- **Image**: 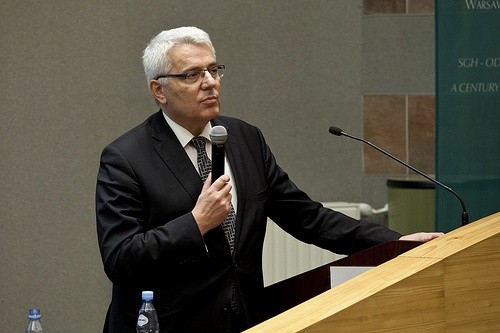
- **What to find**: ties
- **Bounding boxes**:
[188,135,242,315]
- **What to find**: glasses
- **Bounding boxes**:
[155,63,225,83]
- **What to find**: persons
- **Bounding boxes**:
[95,26,445,333]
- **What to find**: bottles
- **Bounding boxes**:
[137,291,160,333]
[24,309,43,333]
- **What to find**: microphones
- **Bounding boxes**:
[329,126,469,226]
[209,125,228,187]
